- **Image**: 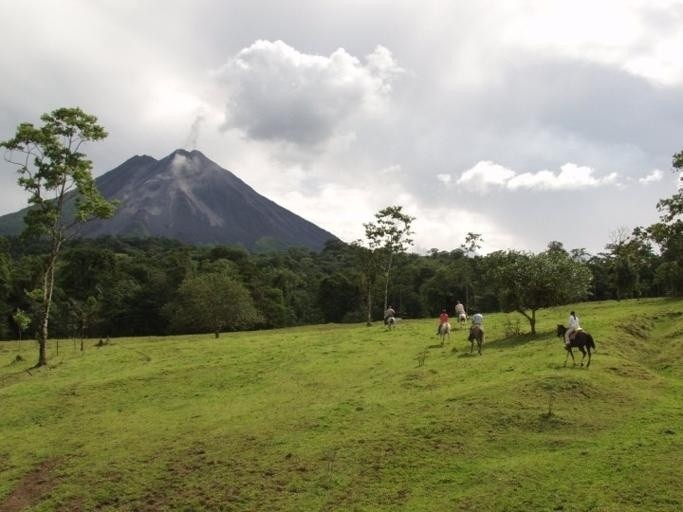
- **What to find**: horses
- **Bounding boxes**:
[556,323,595,368]
[439,322,451,347]
[384,310,395,330]
[458,313,466,330]
[468,318,484,355]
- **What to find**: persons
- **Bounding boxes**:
[455,299,465,322]
[438,309,449,333]
[384,305,395,320]
[565,310,579,344]
[473,311,483,325]
